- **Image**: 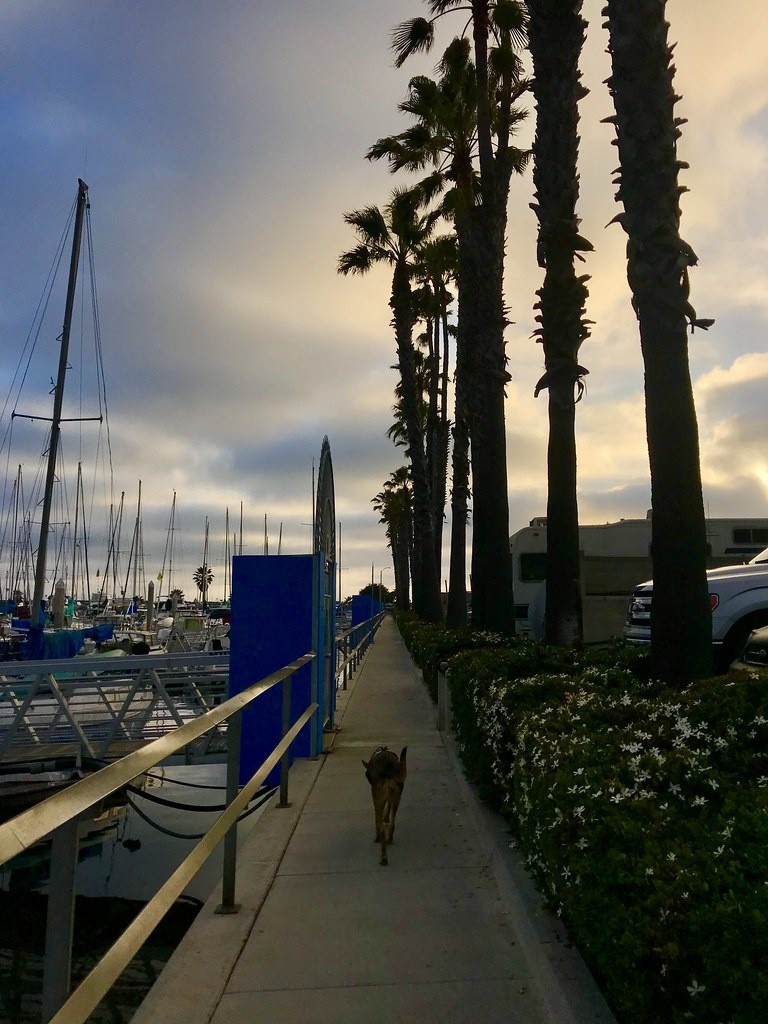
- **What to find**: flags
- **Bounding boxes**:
[96,569,99,577]
[157,572,163,581]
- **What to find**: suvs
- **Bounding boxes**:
[621,545,768,676]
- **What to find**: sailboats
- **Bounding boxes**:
[0,161,230,863]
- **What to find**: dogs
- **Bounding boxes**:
[362,745,408,867]
